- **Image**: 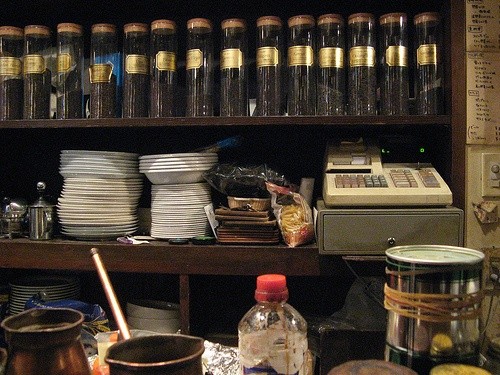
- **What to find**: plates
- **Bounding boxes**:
[56,150,143,239]
[9,279,79,315]
[150,185,215,240]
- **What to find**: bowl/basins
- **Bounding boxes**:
[125,298,180,333]
[138,152,219,184]
[227,196,270,211]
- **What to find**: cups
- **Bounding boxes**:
[26,198,56,240]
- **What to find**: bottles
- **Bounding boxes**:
[381,12,409,118]
[414,12,446,116]
[23,24,51,121]
[286,15,317,116]
[346,12,377,117]
[149,19,179,118]
[314,15,345,118]
[90,24,117,119]
[185,18,215,118]
[219,19,251,117]
[255,15,286,116]
[55,23,85,121]
[0,26,25,121]
[0,307,93,375]
[123,23,148,119]
[104,333,206,375]
[237,274,310,375]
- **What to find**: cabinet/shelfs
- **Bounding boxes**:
[0,0,466,336]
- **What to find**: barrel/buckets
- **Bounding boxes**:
[382,245,486,375]
